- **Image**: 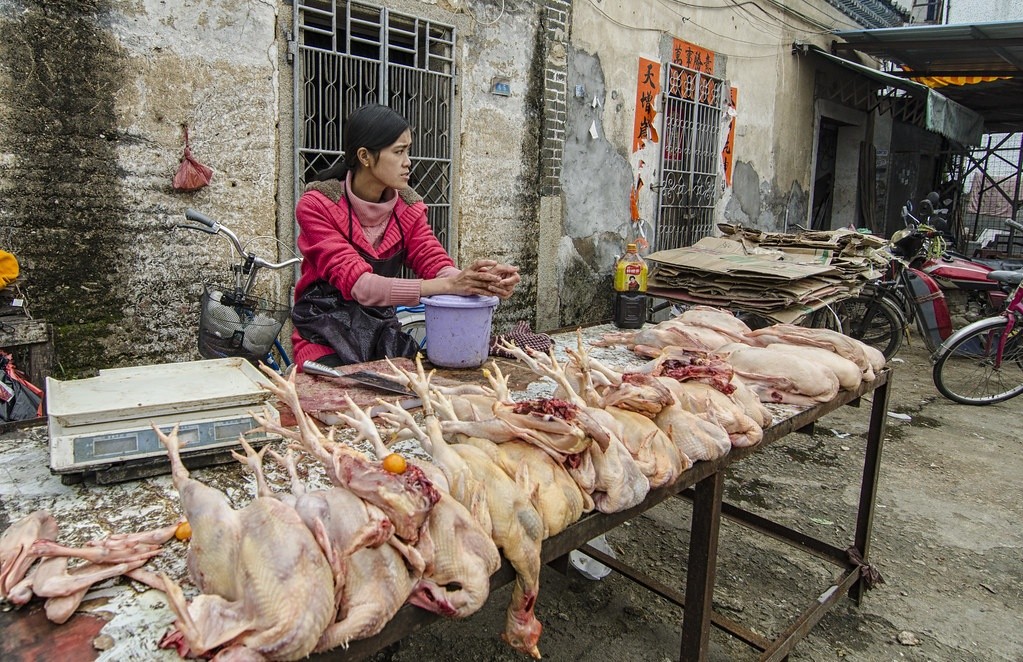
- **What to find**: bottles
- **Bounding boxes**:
[615,243,648,328]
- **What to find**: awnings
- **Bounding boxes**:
[791,39,986,150]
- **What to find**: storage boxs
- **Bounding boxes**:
[419,290,500,372]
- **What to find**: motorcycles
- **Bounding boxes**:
[843,191,1023,361]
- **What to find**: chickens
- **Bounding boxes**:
[148,328,773,658]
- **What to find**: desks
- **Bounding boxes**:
[0,311,893,662]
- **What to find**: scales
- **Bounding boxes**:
[45,356,283,486]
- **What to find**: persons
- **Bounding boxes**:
[287,105,523,375]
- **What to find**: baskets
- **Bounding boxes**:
[197,284,291,369]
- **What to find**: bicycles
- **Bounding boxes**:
[177,207,428,375]
[928,217,1023,405]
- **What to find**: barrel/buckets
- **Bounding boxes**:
[420,292,498,368]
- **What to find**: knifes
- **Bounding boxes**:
[302,360,418,399]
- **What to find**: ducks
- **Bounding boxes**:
[767,340,862,392]
[743,323,886,382]
[587,321,727,359]
[712,342,841,406]
[673,304,753,347]
